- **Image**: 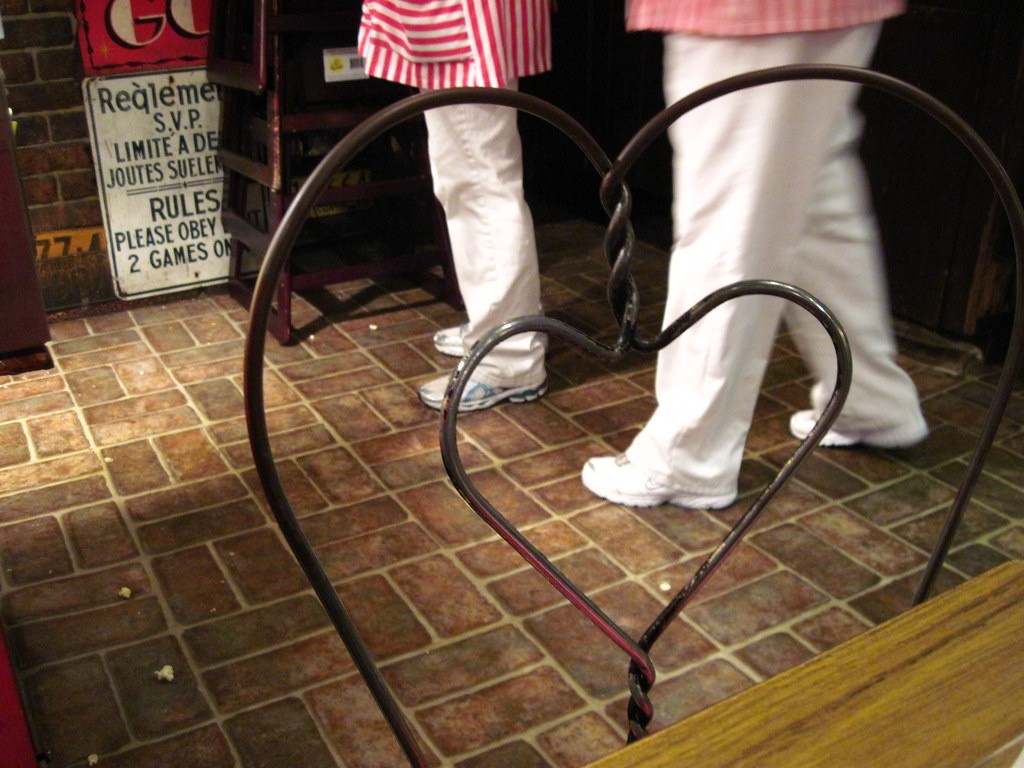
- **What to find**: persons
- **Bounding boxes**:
[581,0,930,510]
[358,0,552,412]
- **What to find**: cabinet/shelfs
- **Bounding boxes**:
[208,0,464,345]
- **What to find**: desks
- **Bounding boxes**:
[596,560,1024,768]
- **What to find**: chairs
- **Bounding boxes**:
[245,61,1024,768]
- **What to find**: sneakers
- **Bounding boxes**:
[420,369,548,413]
[790,409,930,449]
[581,454,737,510]
[434,322,469,357]
